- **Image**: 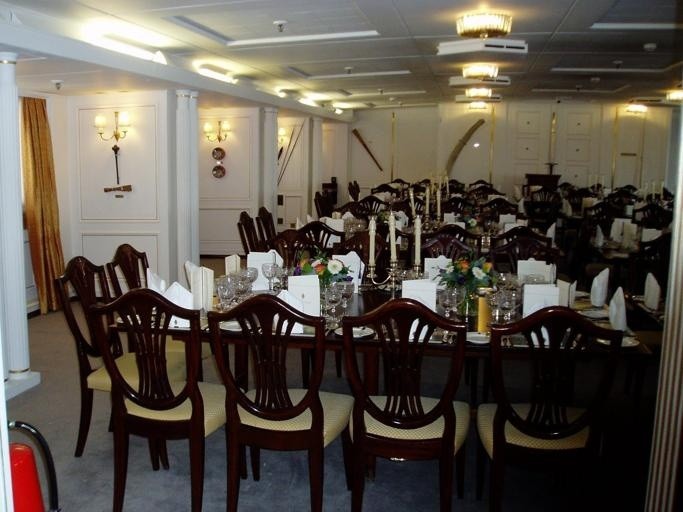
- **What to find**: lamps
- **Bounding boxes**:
[203,120,230,143]
[463,63,498,109]
[94,113,130,155]
[455,12,513,40]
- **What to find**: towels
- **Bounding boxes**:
[162,282,194,328]
[146,268,165,296]
[185,260,214,311]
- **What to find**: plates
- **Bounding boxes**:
[218,282,638,347]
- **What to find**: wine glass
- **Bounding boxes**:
[215,261,547,346]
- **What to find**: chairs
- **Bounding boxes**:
[106,244,212,359]
[53,255,187,469]
[86,288,245,512]
[342,297,473,512]
[206,293,355,511]
[7,420,63,512]
[473,305,623,512]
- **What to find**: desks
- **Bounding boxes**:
[525,173,561,193]
